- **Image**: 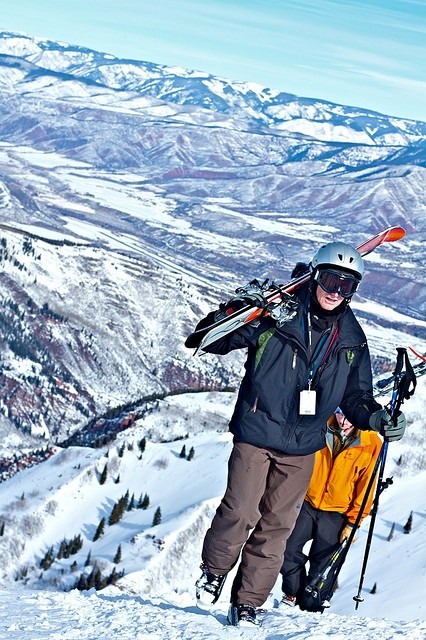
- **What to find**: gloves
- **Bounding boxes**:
[368,408,406,442]
[213,289,269,322]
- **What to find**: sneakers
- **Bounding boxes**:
[195,562,227,607]
[277,594,297,610]
[227,603,264,627]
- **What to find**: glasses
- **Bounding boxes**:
[309,262,361,298]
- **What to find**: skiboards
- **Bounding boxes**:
[184,226,406,356]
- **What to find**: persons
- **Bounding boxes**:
[195,242,407,630]
[279,407,383,615]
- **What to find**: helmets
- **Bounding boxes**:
[308,242,365,281]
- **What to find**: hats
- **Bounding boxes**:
[333,407,344,416]
